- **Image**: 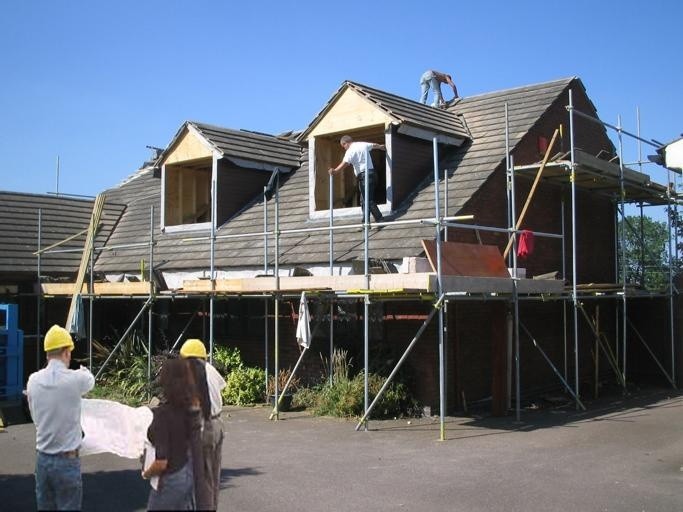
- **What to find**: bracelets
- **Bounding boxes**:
[141,472,149,480]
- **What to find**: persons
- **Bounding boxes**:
[418,70,459,111]
[328,134,386,232]
[26,324,95,511]
[182,359,211,435]
[179,338,226,512]
[139,357,203,511]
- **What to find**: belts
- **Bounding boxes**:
[50,450,80,457]
[211,414,221,419]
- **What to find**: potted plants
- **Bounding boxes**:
[268,369,301,412]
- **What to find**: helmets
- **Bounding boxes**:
[44,324,75,353]
[180,338,206,358]
